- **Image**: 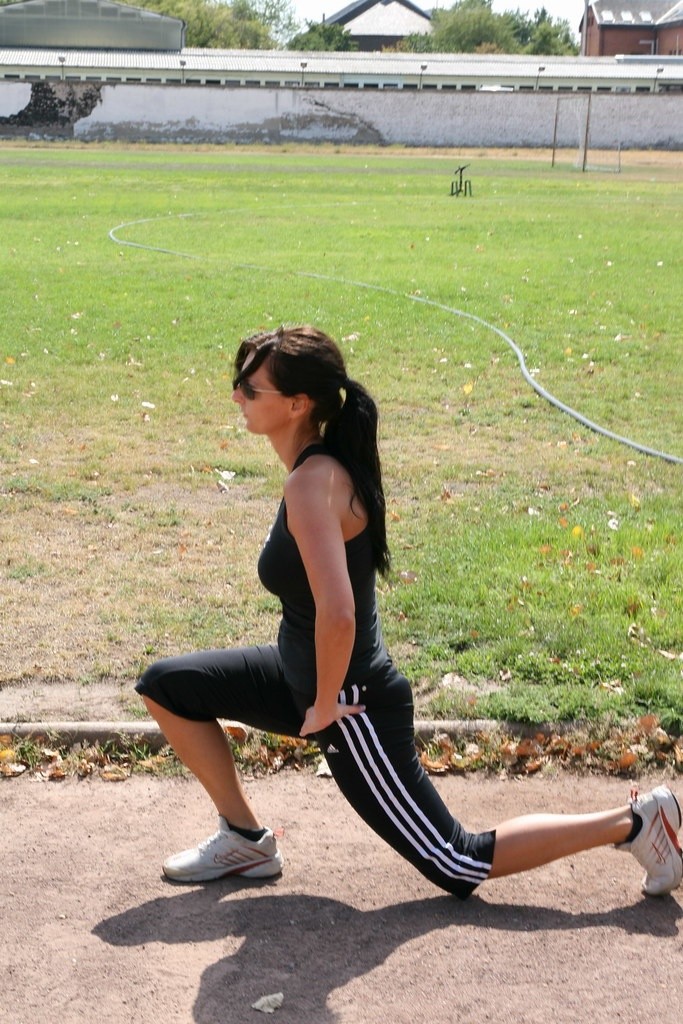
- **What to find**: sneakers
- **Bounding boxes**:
[162,815,284,881]
[615,785,683,896]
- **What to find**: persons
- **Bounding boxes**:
[135,328,683,896]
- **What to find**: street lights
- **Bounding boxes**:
[417,62,429,91]
[534,66,545,91]
[300,61,307,88]
[58,56,68,81]
[179,60,187,84]
[651,67,664,95]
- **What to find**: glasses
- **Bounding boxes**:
[239,380,282,400]
